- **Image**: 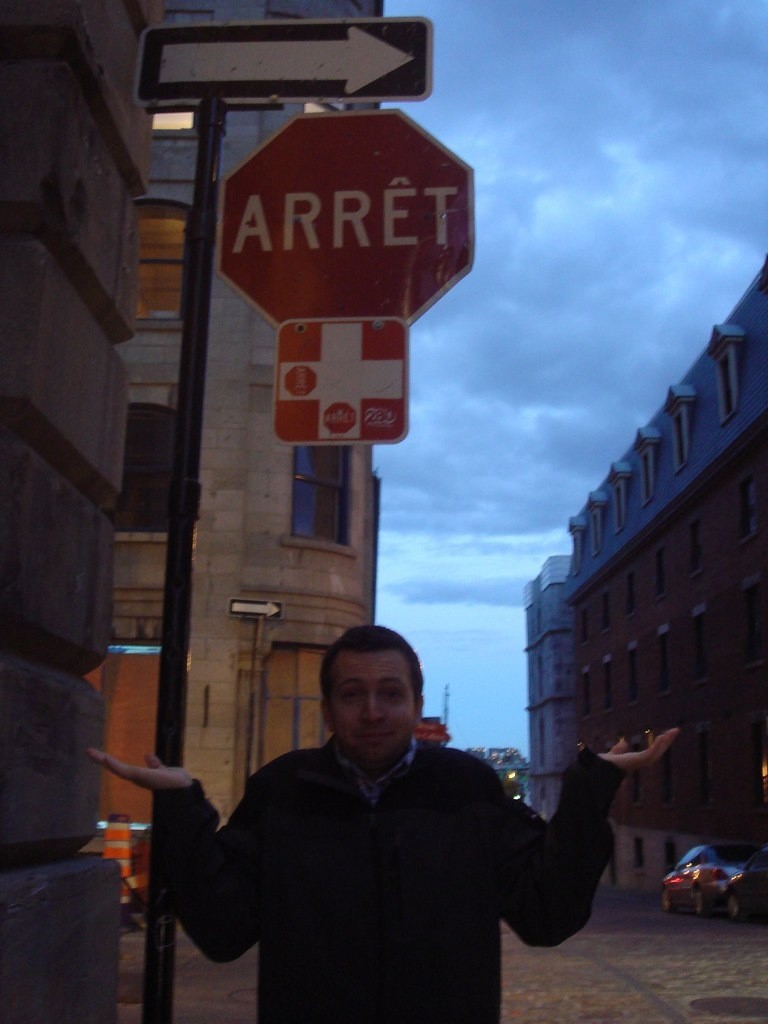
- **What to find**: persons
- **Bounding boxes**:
[84,624,678,1024]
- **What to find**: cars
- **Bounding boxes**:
[720,846,768,923]
[661,842,760,917]
